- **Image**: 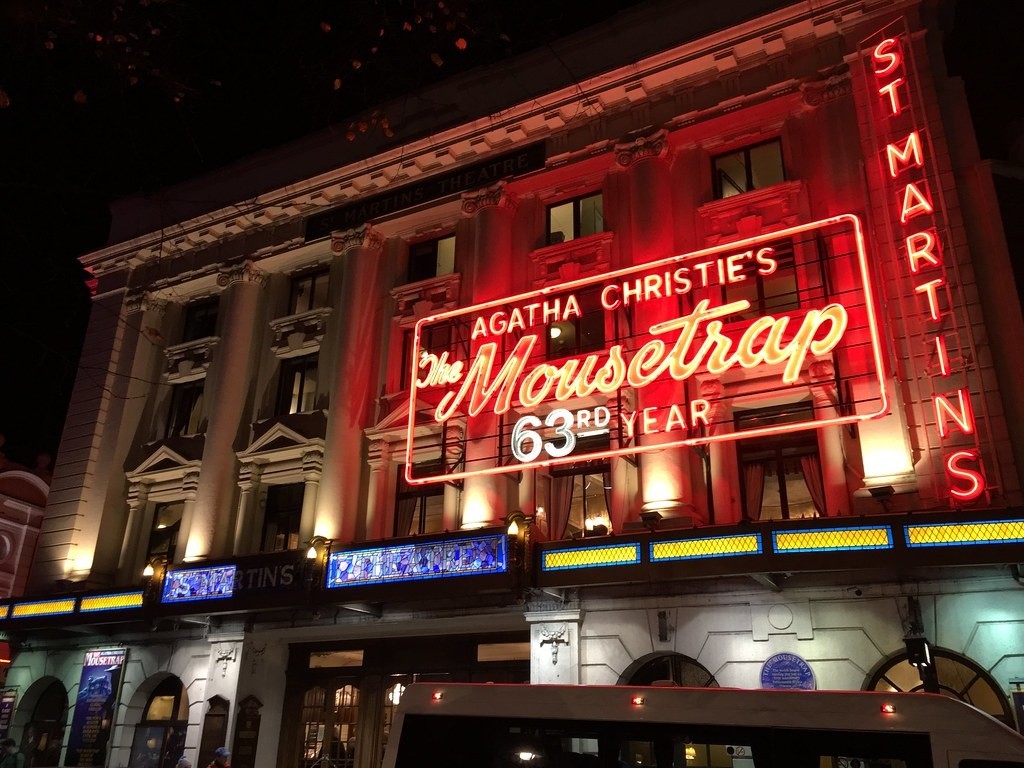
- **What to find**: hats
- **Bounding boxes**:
[215,747,231,756]
[178,755,192,768]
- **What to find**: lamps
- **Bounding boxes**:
[868,484,895,511]
[305,536,328,565]
[507,512,523,562]
[640,511,663,529]
[901,634,931,682]
[142,557,163,576]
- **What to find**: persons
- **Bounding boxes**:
[176,756,192,768]
[20,727,61,768]
[316,727,388,768]
[0,738,25,768]
[207,747,231,768]
